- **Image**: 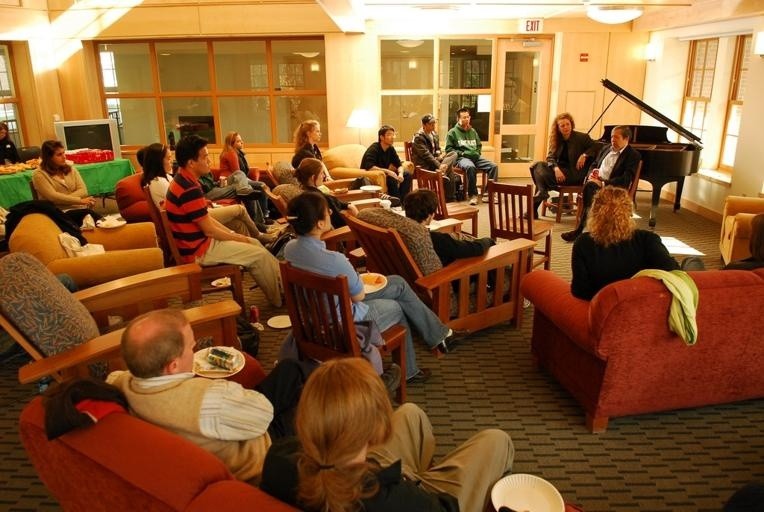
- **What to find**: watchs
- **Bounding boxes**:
[602,181,605,187]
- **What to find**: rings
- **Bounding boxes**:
[559,178,560,180]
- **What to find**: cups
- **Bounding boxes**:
[219,176,224,188]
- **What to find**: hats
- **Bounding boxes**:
[422,115,439,124]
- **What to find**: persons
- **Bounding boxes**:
[519,113,599,218]
[2,107,514,511]
[561,124,640,241]
[570,185,681,301]
[681,214,764,272]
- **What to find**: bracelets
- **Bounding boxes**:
[582,153,587,158]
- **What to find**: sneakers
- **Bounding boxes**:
[482,193,498,204]
[379,363,400,391]
[469,195,478,205]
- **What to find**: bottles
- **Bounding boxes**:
[64,147,114,164]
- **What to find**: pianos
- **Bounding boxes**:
[585,79,704,231]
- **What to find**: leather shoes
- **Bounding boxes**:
[519,211,538,220]
[438,329,469,353]
[407,368,432,383]
[561,230,576,240]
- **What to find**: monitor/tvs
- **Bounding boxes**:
[53,119,122,161]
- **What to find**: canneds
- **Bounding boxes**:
[207,349,237,370]
[592,169,599,179]
[249,306,260,323]
[219,176,225,188]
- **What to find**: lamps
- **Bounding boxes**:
[752,30,764,57]
[646,42,656,62]
[583,4,644,26]
[346,109,373,143]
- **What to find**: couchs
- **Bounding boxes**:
[16,388,587,511]
[516,259,761,435]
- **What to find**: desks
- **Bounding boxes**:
[658,233,707,257]
[0,158,134,210]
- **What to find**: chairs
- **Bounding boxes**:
[575,159,643,227]
[278,258,411,403]
[486,178,553,273]
[8,214,167,286]
[0,247,241,385]
[542,141,602,221]
[718,193,764,265]
[113,143,413,226]
[414,165,486,239]
[142,183,247,317]
[320,225,358,258]
[338,210,538,361]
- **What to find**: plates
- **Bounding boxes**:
[193,346,246,379]
[210,277,231,287]
[267,314,292,329]
[360,273,388,294]
[491,472,566,512]
[97,220,128,229]
[360,185,383,192]
